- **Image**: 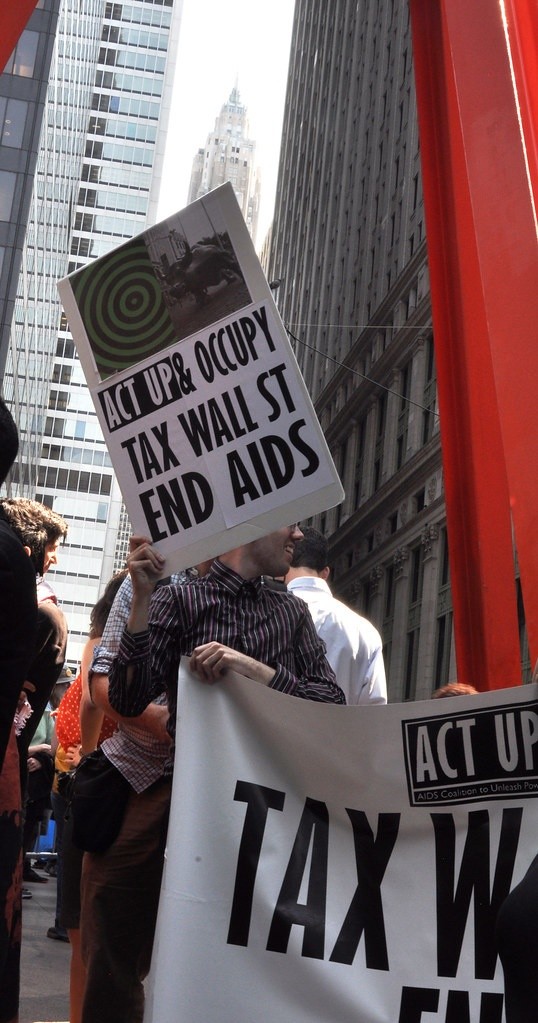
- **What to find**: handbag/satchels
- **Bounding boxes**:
[63,749,131,850]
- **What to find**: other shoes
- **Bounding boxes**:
[22,888,32,898]
[47,927,71,945]
[22,867,49,883]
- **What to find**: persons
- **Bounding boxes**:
[48,560,130,1023]
[42,667,75,718]
[109,524,349,758]
[0,498,66,828]
[0,401,37,778]
[22,708,56,882]
[279,527,388,705]
[81,555,214,1023]
[20,498,67,663]
